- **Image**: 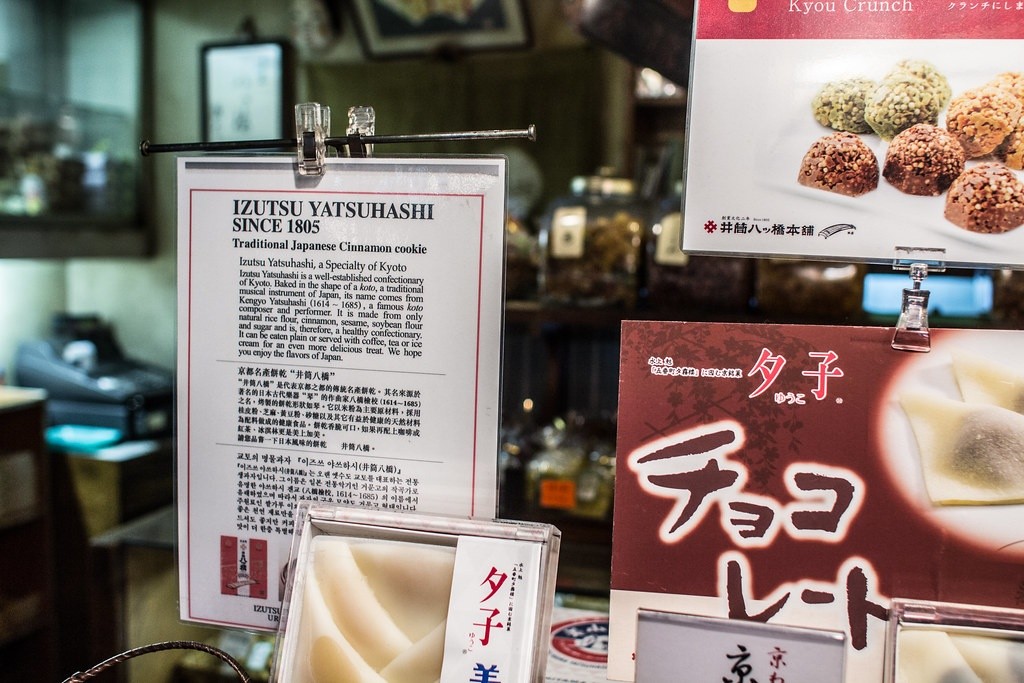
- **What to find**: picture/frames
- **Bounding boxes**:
[346,0,539,62]
[197,36,296,142]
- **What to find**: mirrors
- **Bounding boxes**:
[0,0,159,265]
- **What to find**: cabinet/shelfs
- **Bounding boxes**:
[0,384,60,683]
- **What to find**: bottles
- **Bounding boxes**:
[539,173,642,308]
[647,182,758,306]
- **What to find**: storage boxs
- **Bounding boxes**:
[68,438,173,532]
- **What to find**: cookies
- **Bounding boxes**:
[797,58,1024,234]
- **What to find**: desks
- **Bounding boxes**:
[506,297,856,418]
[87,508,177,683]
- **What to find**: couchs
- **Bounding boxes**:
[17,312,175,439]
